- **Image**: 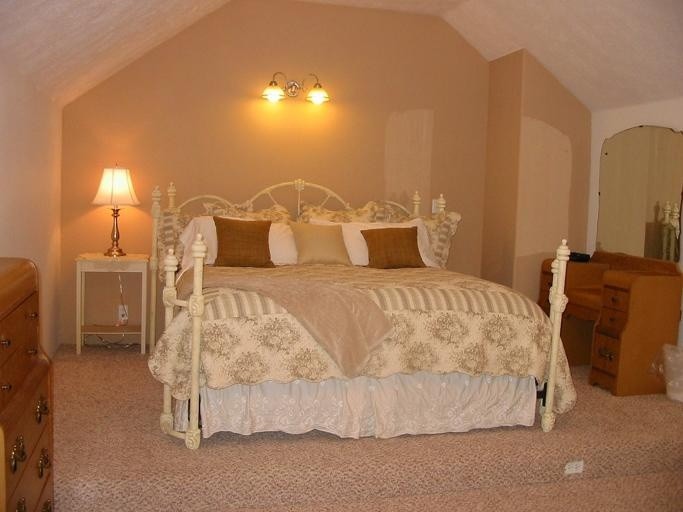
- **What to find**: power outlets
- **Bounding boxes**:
[118,305,128,320]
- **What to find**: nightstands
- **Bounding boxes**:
[75,254,150,355]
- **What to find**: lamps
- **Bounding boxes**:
[91,161,141,256]
[261,72,331,105]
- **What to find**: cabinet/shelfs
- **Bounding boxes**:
[0,258,54,512]
[537,249,683,396]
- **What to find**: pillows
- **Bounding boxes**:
[180,214,436,267]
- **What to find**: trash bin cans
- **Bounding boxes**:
[661,344,683,403]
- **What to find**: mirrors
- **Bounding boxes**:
[596,125,683,266]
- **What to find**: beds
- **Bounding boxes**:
[150,179,577,449]
